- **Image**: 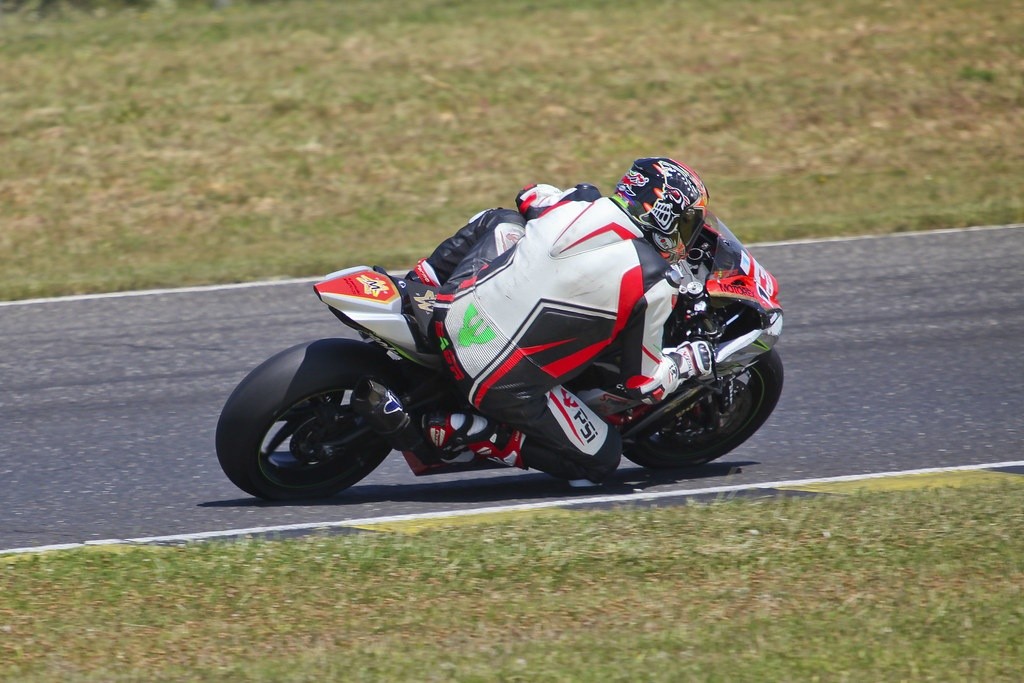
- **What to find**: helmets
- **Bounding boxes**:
[610,156,709,264]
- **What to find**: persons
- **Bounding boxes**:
[406,156,715,492]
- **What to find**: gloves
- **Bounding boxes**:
[666,337,719,384]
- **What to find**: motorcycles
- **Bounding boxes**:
[212,204,786,502]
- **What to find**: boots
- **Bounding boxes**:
[421,408,529,472]
[405,257,440,287]
